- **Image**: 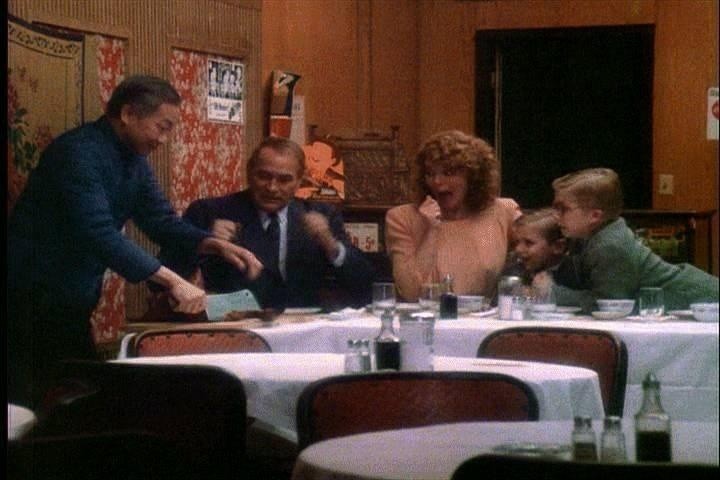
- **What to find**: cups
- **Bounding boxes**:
[372,281,395,319]
[639,287,665,320]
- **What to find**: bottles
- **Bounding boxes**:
[344,305,434,373]
[573,370,671,461]
[512,296,529,321]
[497,275,522,320]
[440,272,458,320]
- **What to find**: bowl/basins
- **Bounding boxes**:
[690,303,720,322]
[455,294,485,312]
[597,299,635,317]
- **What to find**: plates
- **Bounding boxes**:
[591,311,625,320]
[279,307,321,315]
[492,442,566,460]
[667,310,693,317]
[530,304,582,318]
[377,302,423,311]
[331,306,366,319]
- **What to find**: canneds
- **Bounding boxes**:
[344,339,371,375]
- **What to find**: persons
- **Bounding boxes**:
[384,130,526,302]
[1,75,265,437]
[294,138,346,199]
[146,135,373,323]
[489,207,585,307]
[531,166,720,314]
[209,62,242,99]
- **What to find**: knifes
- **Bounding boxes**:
[174,289,263,323]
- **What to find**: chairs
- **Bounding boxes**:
[451,453,720,479]
[296,373,538,448]
[7,362,248,480]
[127,325,271,357]
[477,327,627,416]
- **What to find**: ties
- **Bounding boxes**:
[265,210,280,273]
